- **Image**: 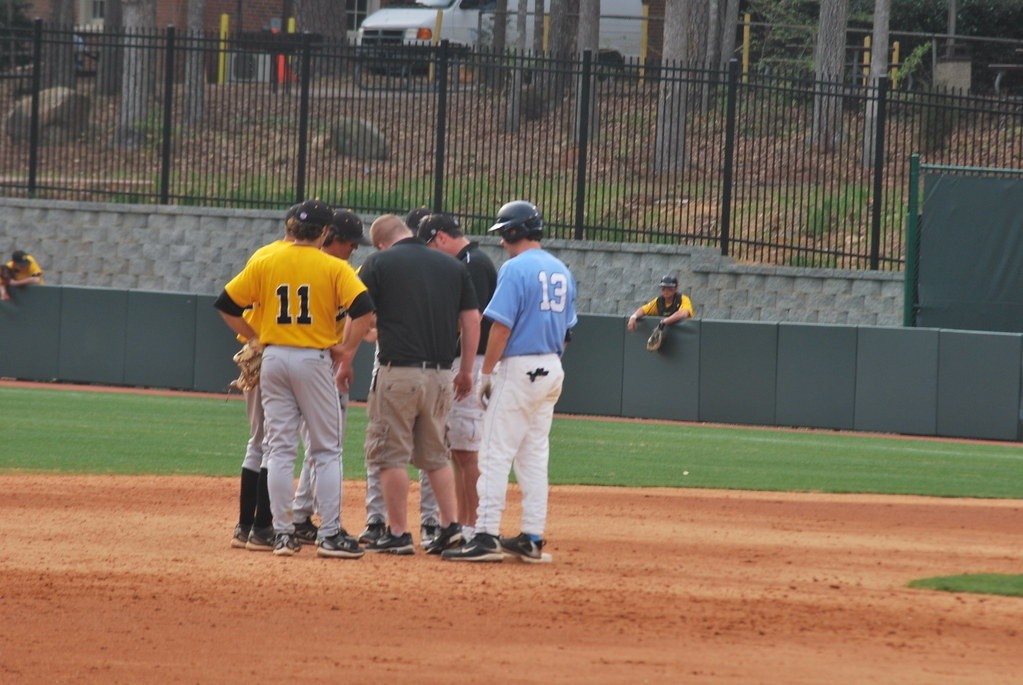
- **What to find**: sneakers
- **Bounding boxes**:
[421,524,440,547]
[272,533,301,555]
[293,516,318,544]
[359,521,385,543]
[501,532,544,561]
[231,522,250,547]
[364,525,415,554]
[425,522,466,554]
[442,533,503,562]
[245,523,276,552]
[316,529,364,559]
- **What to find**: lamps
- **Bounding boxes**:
[222,52,272,84]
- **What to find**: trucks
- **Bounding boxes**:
[355,0,643,83]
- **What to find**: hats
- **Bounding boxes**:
[12,251,32,266]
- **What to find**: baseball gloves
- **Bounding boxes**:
[224,334,263,403]
[646,320,665,352]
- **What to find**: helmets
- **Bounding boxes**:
[487,200,538,233]
[295,201,332,224]
[417,214,453,245]
[406,207,432,236]
[332,209,371,247]
[286,203,301,224]
[659,275,677,287]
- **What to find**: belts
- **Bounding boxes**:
[380,360,451,370]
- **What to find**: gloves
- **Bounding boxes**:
[526,368,548,382]
[476,369,492,410]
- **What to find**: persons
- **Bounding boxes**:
[0,266,12,287]
[627,275,693,350]
[440,202,578,562]
[358,208,439,544]
[214,201,376,561]
[357,214,482,556]
[0,250,44,300]
[417,214,498,550]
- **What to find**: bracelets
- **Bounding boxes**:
[658,321,664,330]
[630,315,637,320]
